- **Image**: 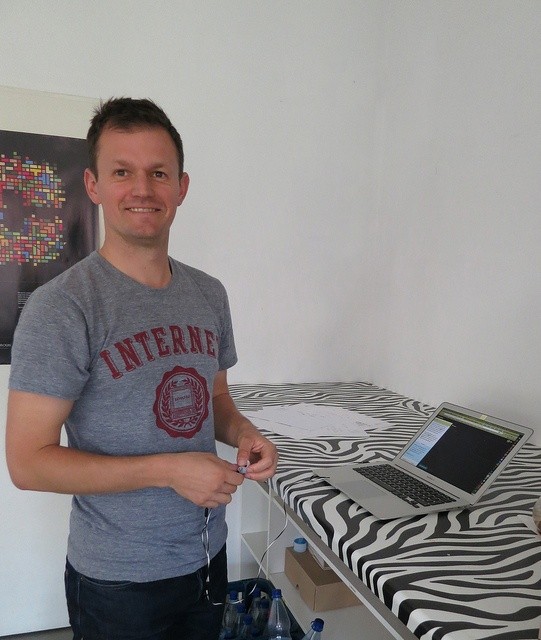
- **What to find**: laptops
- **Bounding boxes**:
[311,401,535,521]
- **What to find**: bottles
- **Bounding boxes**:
[269,589,291,640]
[224,590,243,630]
[234,604,246,640]
[248,588,268,634]
[249,629,264,640]
[219,594,233,640]
[239,615,256,640]
[302,618,324,640]
[257,599,270,640]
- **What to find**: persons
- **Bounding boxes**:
[3,94,281,639]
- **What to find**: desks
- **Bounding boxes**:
[230,375,540,640]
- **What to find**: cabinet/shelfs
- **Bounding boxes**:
[237,448,408,640]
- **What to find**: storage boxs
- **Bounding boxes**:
[284,547,363,613]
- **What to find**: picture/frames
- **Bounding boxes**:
[0,128,99,365]
